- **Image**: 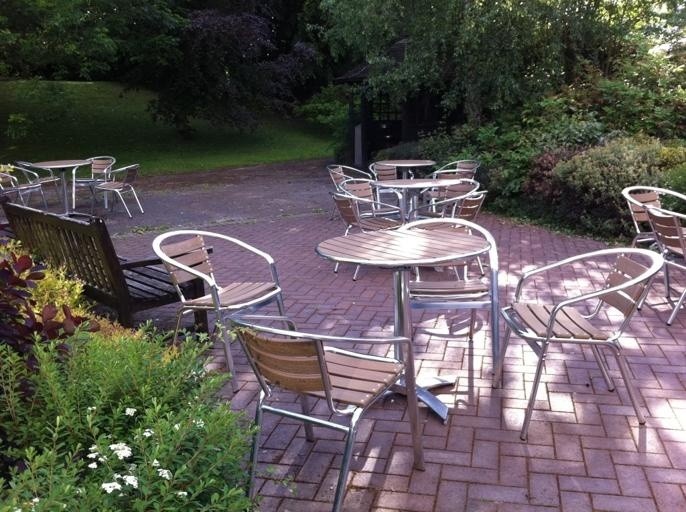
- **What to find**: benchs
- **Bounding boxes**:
[0,195,214,348]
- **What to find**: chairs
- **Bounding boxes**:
[0,156,145,219]
